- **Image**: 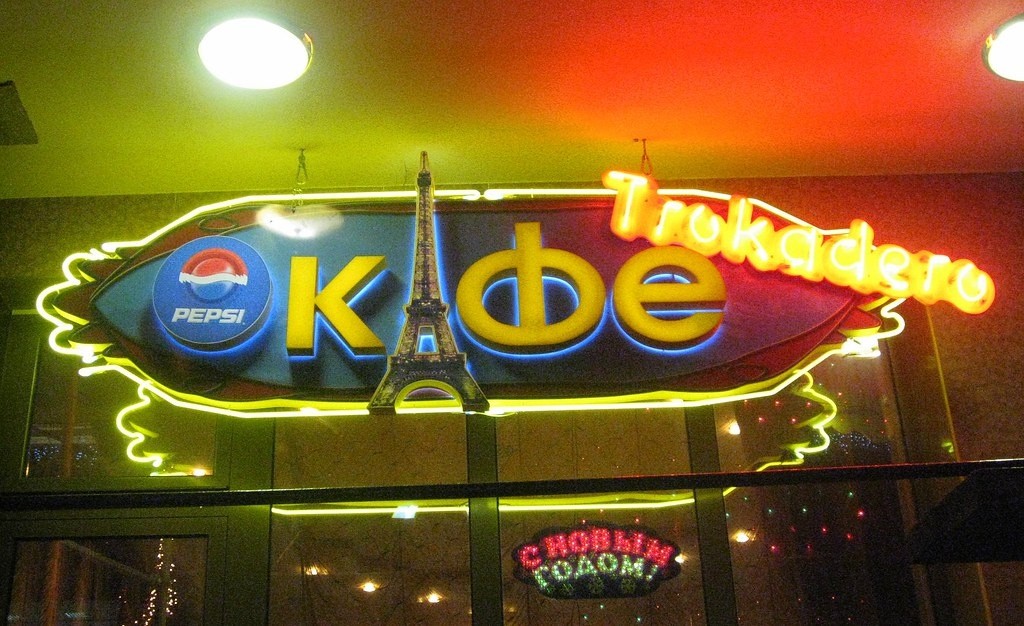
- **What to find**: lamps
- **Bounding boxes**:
[983,13,1024,83]
[197,11,314,90]
